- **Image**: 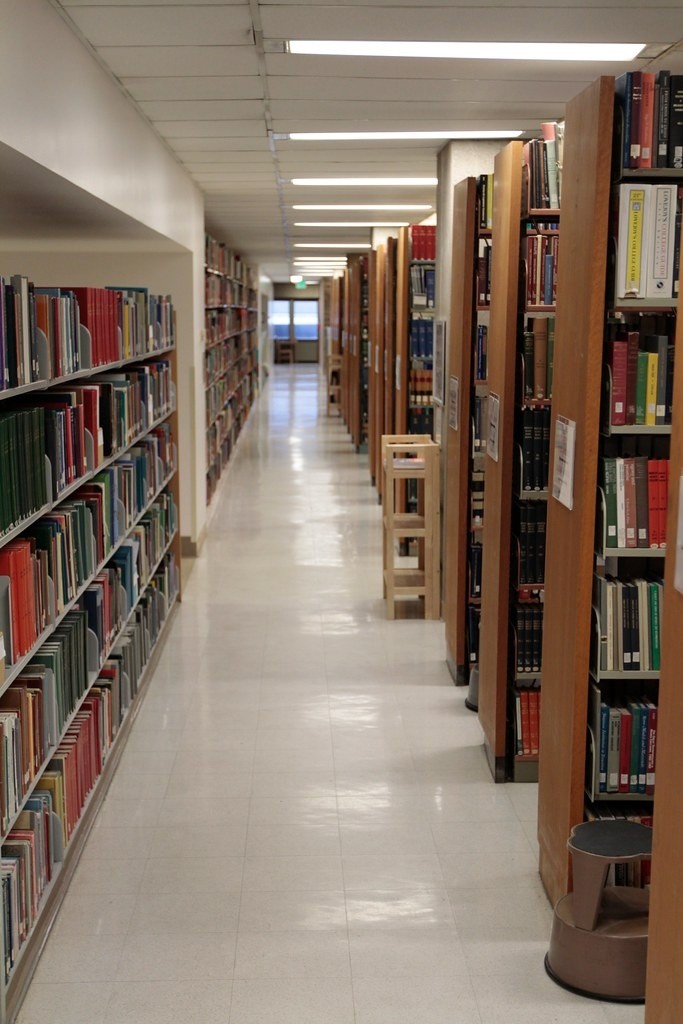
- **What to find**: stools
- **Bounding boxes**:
[545,820,653,1006]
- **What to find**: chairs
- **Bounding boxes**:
[274,337,294,364]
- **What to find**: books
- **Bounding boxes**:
[359,65,683,824]
[0,236,258,983]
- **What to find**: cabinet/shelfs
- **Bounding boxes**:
[323,248,377,487]
[477,119,565,782]
[535,72,683,912]
[0,276,181,1024]
[376,235,398,505]
[444,176,493,686]
[394,225,437,557]
[380,434,439,620]
[203,231,260,505]
